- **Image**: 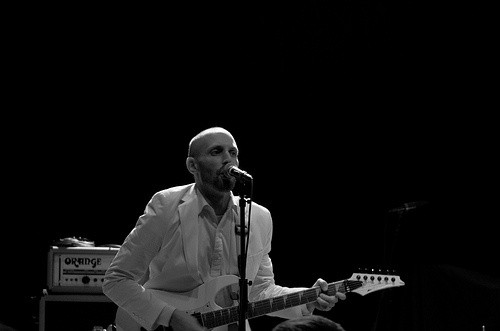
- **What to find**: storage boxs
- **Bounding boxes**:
[38,288,114,331]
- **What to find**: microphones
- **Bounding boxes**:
[228,165,251,180]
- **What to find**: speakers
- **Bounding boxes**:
[38,293,119,331]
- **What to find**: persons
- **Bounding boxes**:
[272,315,344,331]
[101,127,348,331]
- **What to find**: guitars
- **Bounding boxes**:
[115,266,406,331]
